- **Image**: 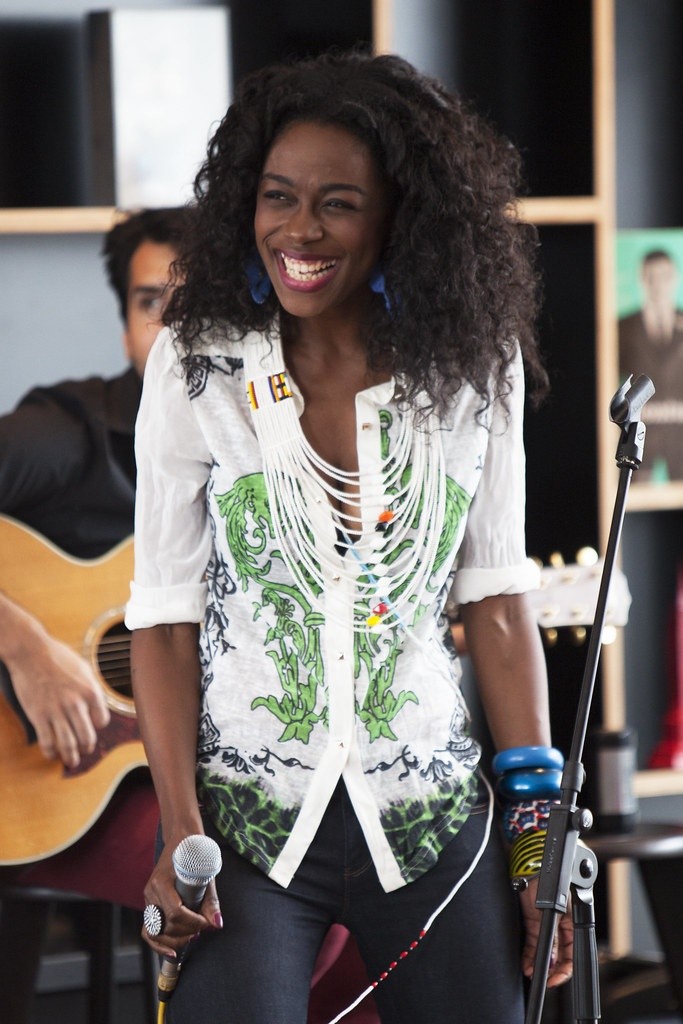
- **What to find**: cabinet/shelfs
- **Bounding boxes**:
[1,0,683,965]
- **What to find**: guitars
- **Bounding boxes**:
[0,508,632,869]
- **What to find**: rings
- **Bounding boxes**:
[141,905,162,935]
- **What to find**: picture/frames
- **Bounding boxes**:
[612,224,683,488]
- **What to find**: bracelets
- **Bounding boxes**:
[488,746,567,882]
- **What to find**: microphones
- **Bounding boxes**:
[156,833,224,1004]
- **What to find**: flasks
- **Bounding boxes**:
[593,725,641,832]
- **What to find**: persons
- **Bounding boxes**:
[0,204,198,780]
[618,245,683,490]
[118,50,586,1024]
